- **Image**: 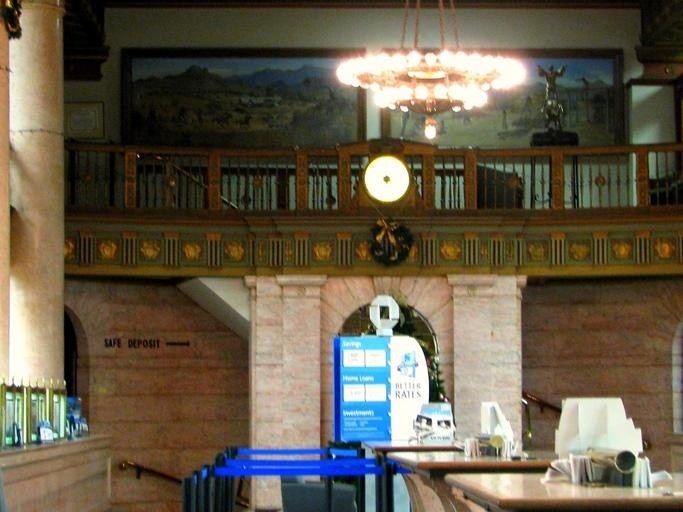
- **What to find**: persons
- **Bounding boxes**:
[538,64,566,109]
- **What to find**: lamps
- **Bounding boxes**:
[336,1,530,141]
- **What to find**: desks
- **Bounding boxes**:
[362,441,682,511]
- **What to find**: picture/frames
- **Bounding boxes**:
[121,47,626,155]
[63,101,104,140]
